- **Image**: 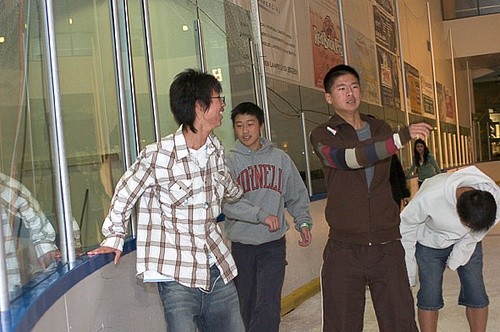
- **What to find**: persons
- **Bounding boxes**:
[308,64,500,332]
[222,102,313,332]
[86,67,246,332]
[0,169,112,295]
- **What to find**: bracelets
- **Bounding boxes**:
[300,223,312,230]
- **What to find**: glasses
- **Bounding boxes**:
[209,96,226,103]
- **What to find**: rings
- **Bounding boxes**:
[416,134,421,140]
